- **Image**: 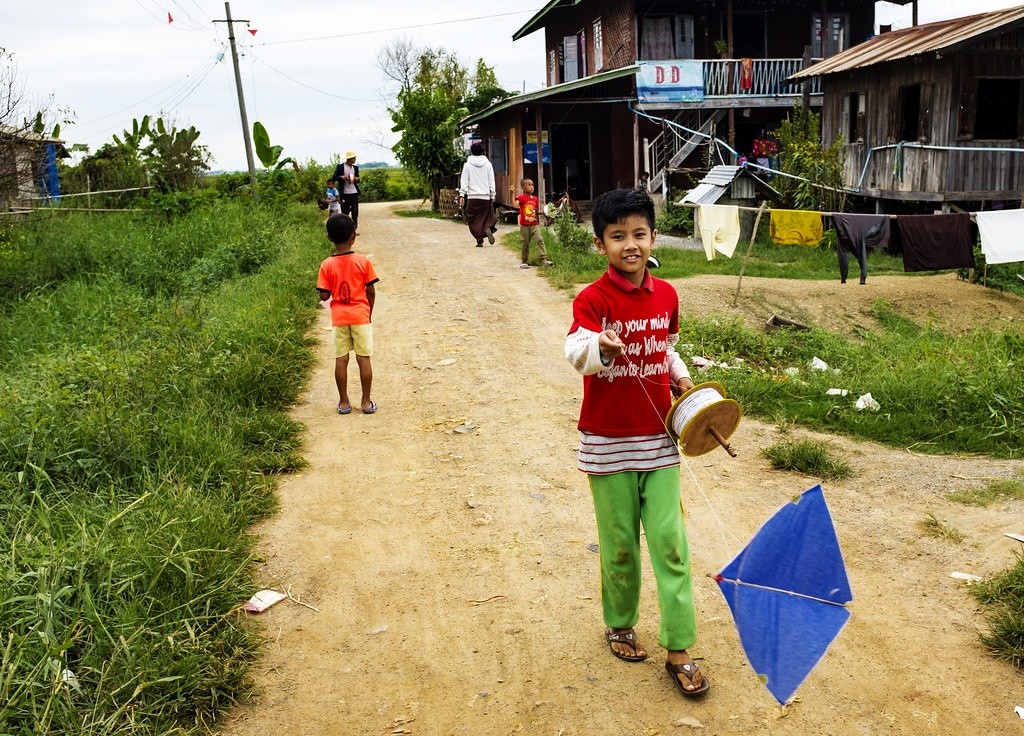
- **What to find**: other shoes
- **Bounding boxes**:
[476,244,484,247]
[485,228,495,244]
[520,264,528,269]
[355,232,360,236]
[545,261,553,266]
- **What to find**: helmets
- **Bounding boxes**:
[344,149,358,159]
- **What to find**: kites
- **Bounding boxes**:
[714,485,853,705]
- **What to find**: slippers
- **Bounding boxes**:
[338,400,352,413]
[604,627,646,662]
[362,400,378,413]
[664,658,710,695]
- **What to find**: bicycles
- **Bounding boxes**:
[543,187,581,227]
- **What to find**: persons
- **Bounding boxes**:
[332,150,361,236]
[564,189,710,694]
[326,178,339,218]
[510,178,554,269]
[639,172,649,187]
[316,213,380,414]
[459,143,498,247]
